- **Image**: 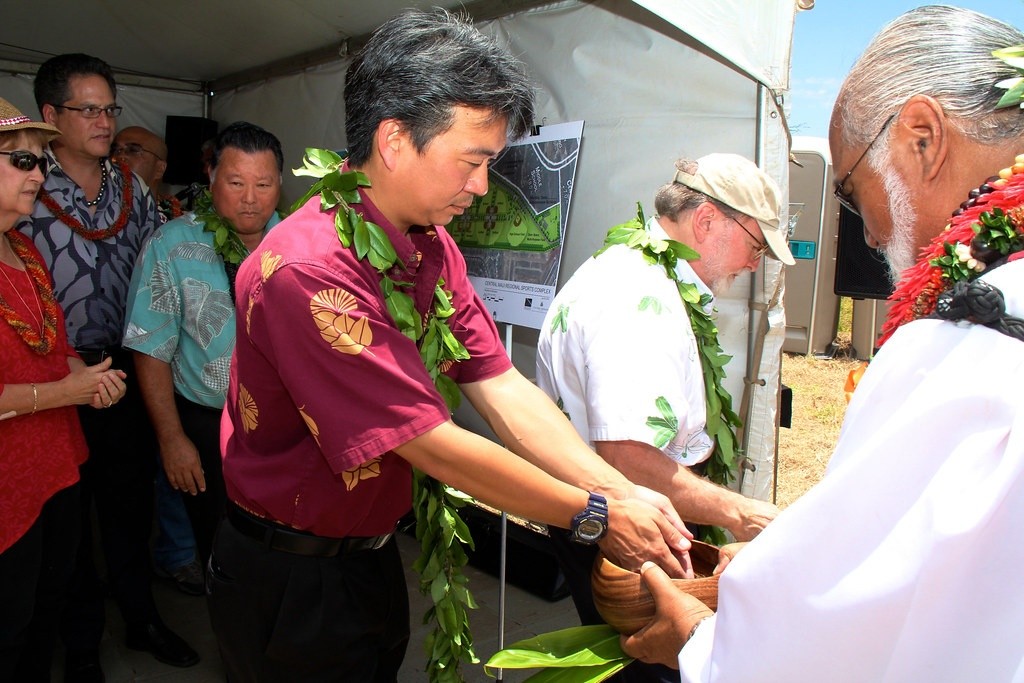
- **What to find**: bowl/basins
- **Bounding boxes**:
[591,539,734,638]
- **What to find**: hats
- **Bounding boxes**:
[0,98,63,144]
[674,152,797,267]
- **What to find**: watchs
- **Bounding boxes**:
[570,491,608,547]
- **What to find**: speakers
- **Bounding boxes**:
[833,201,900,302]
[163,116,219,184]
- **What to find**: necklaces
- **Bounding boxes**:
[592,200,746,547]
[285,146,482,682]
[194,189,250,309]
[86,156,106,206]
[871,153,1024,352]
[0,227,58,357]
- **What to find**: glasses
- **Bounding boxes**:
[730,217,769,260]
[833,115,898,219]
[61,104,123,119]
[1,151,48,177]
[111,142,162,162]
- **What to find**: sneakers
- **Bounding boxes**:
[157,554,204,596]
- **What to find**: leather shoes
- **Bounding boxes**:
[64,649,104,683]
[127,618,201,668]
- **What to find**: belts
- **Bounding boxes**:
[227,500,392,559]
[77,346,130,364]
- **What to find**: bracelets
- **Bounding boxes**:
[687,613,714,641]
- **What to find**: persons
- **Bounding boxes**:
[535,153,797,629]
[16,51,201,683]
[1,97,129,682]
[121,121,289,585]
[619,5,1024,683]
[205,0,694,683]
